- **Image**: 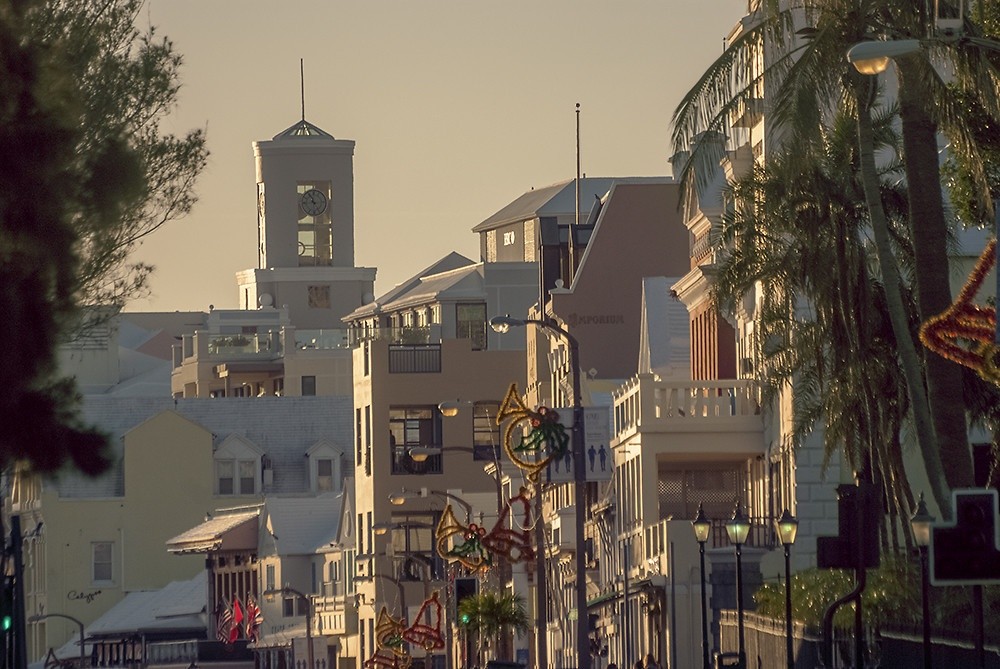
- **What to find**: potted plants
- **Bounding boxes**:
[211,336,233,354]
[230,334,251,354]
[397,323,432,346]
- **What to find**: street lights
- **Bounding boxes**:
[487,314,593,669]
[773,508,799,669]
[263,587,316,669]
[28,613,86,669]
[387,485,486,669]
[724,500,752,669]
[439,398,550,669]
[372,518,455,669]
[409,445,510,665]
[690,501,713,669]
[350,549,434,669]
[352,575,412,669]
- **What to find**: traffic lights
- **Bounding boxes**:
[932,488,1000,585]
[452,575,481,630]
[0,574,14,633]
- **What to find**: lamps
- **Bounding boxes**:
[258,292,275,310]
[362,291,375,305]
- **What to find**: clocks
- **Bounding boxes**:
[299,188,329,218]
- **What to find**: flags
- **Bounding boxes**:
[245,597,264,646]
[216,597,243,644]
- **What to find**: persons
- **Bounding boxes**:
[390,429,396,472]
